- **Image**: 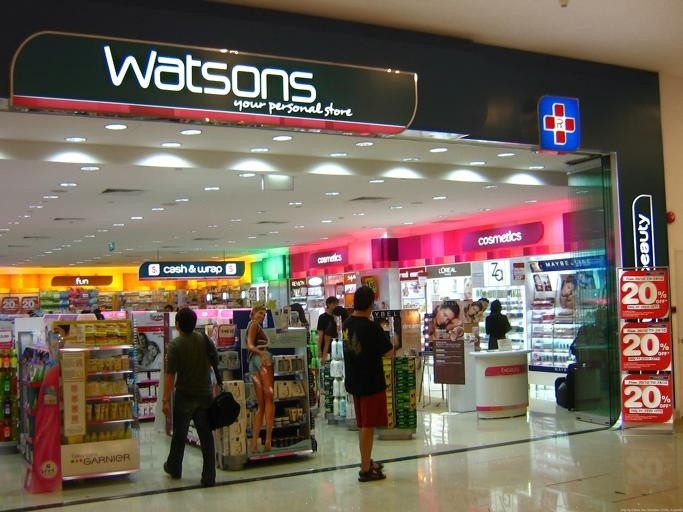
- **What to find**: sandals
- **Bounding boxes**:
[358,459,386,481]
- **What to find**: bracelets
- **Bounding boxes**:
[162,399,170,401]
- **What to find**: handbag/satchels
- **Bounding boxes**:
[205,392,240,431]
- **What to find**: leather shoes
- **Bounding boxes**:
[164,462,182,478]
[201,476,215,485]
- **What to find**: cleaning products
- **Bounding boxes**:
[86,430,132,442]
[29,358,44,381]
[280,382,290,396]
[292,380,306,397]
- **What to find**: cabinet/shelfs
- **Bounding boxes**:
[135,370,160,420]
[22,345,140,481]
[240,328,312,458]
[114,290,173,312]
[529,270,606,374]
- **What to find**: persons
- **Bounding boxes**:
[533,274,544,291]
[161,307,224,487]
[164,304,173,312]
[288,303,310,345]
[560,275,577,308]
[136,332,162,370]
[320,306,349,366]
[341,284,395,483]
[316,296,340,360]
[433,301,463,341]
[478,298,489,313]
[485,299,512,350]
[463,301,483,323]
[244,305,275,452]
